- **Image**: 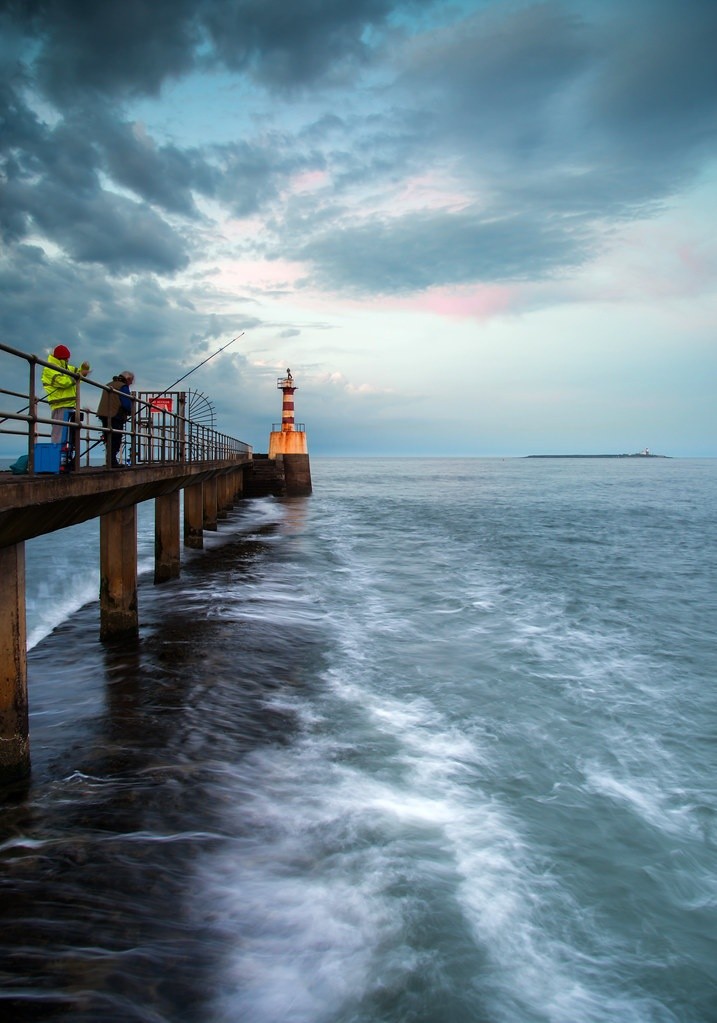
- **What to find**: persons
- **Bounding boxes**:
[41,345,89,443]
[96,371,134,468]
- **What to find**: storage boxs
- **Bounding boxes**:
[34,411,77,473]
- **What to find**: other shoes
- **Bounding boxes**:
[111,463,125,468]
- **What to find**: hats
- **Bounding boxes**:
[54,345,70,359]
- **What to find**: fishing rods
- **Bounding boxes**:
[0,369,95,424]
[80,332,245,458]
